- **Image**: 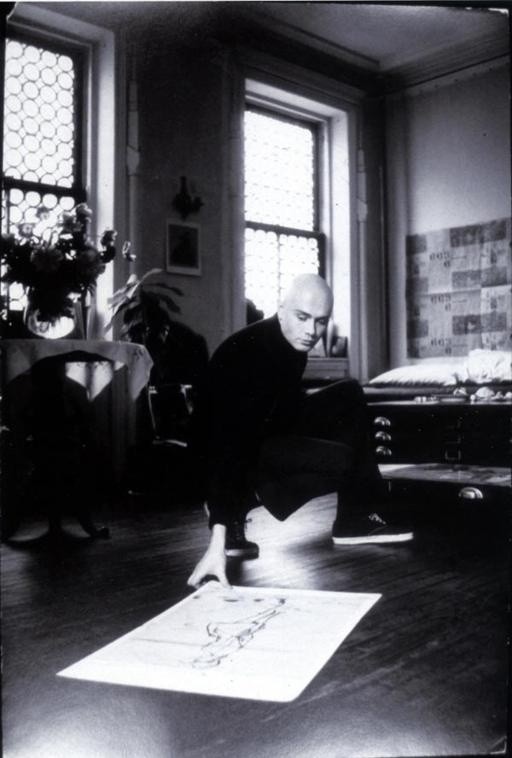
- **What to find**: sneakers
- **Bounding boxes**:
[331,512,414,545]
[204,500,259,557]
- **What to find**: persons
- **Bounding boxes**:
[184,272,419,590]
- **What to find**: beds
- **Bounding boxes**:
[360,347,512,401]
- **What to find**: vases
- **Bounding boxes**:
[20,283,78,339]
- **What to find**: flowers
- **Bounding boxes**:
[0,198,137,289]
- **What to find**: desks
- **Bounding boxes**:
[365,394,512,505]
[1,335,153,544]
